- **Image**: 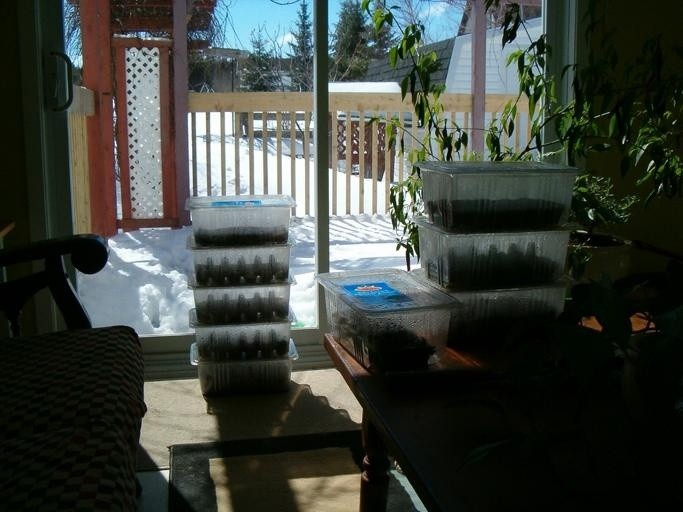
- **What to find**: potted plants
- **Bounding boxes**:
[563,171,641,286]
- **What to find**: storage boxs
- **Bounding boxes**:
[312,267,461,376]
[404,158,576,346]
[179,192,300,399]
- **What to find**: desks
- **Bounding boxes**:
[322,331,683,511]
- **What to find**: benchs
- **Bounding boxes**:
[0,231,165,510]
[331,119,397,182]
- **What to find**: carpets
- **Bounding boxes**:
[135,364,370,478]
[163,429,422,510]
